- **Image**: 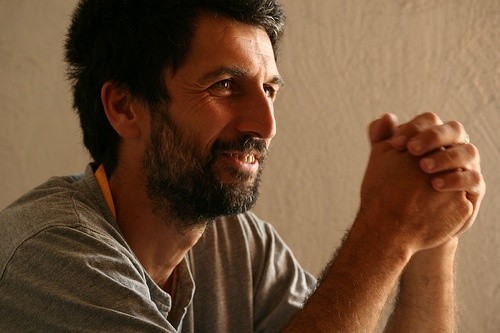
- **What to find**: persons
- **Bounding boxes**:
[0,1,488,333]
